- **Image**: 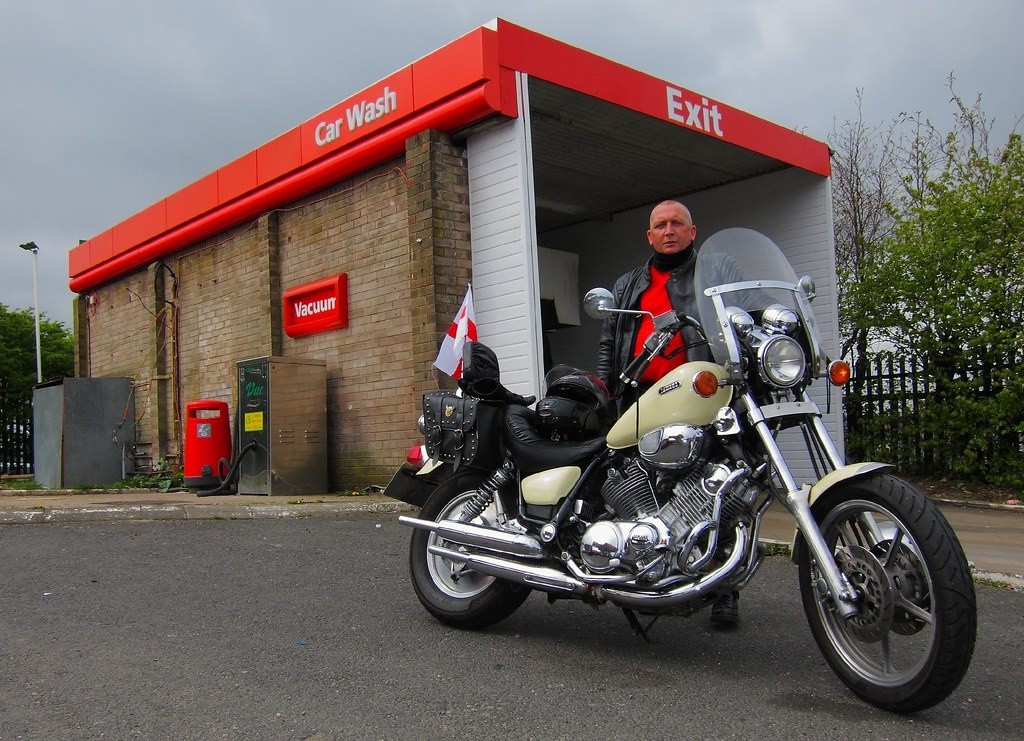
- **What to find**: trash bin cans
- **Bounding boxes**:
[182,400,232,494]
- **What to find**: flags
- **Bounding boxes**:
[432,289,478,380]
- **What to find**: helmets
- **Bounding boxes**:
[536,373,612,442]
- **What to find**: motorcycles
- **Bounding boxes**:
[380,228,977,713]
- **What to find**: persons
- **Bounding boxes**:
[596,200,783,625]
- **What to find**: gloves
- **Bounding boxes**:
[470,377,535,406]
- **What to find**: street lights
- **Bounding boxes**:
[19,241,42,384]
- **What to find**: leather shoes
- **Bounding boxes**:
[710,591,739,623]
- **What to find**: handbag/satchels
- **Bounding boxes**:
[422,393,503,470]
